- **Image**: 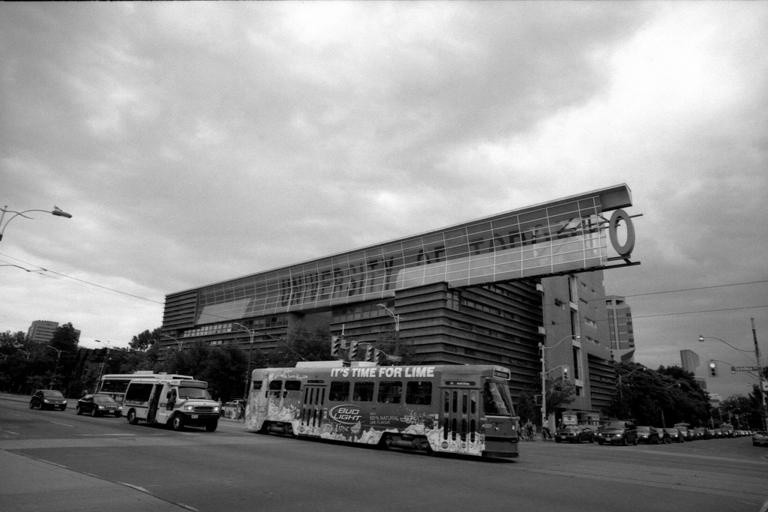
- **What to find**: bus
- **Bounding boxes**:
[95,372,194,418]
[242,360,521,462]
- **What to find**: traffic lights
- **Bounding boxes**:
[365,346,374,363]
[375,348,387,364]
[562,365,570,384]
[348,340,360,361]
[331,335,342,356]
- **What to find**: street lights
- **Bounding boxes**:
[161,334,183,355]
[698,334,767,434]
[46,345,62,387]
[0,205,73,244]
[376,304,400,356]
[234,322,255,400]
[94,339,110,393]
[619,367,646,399]
[540,335,580,422]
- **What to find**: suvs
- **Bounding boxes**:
[597,422,637,446]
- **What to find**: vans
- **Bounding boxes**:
[221,401,243,417]
[121,379,221,433]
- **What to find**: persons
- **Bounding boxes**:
[541,416,552,441]
[524,425,535,441]
[526,424,534,442]
[237,401,247,420]
[515,421,524,440]
[556,418,562,431]
[216,397,223,416]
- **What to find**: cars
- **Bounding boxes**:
[637,423,738,444]
[29,391,67,410]
[554,425,595,443]
[752,431,768,446]
[76,392,122,419]
